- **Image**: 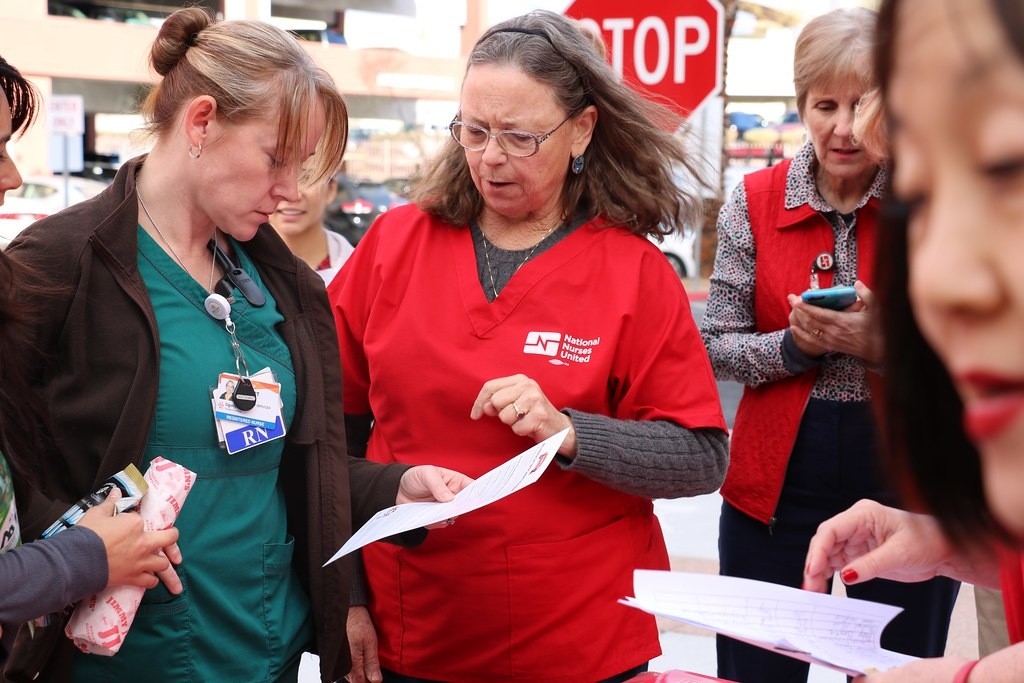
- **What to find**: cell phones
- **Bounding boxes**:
[802,286,858,311]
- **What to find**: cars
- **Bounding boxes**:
[0,177,109,254]
[647,223,698,279]
[324,175,411,247]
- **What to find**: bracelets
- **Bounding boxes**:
[953,660,979,683]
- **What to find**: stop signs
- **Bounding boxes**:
[561,1,723,137]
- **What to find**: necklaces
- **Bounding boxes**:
[479,217,562,298]
[134,174,217,294]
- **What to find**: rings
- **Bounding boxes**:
[810,329,823,340]
[513,403,525,420]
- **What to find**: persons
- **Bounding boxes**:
[328,9,730,683]
[268,155,355,288]
[802,498,1024,683]
[694,10,962,683]
[0,7,476,683]
[0,58,179,626]
[843,0,1024,561]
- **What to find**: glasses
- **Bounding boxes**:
[449,98,588,159]
